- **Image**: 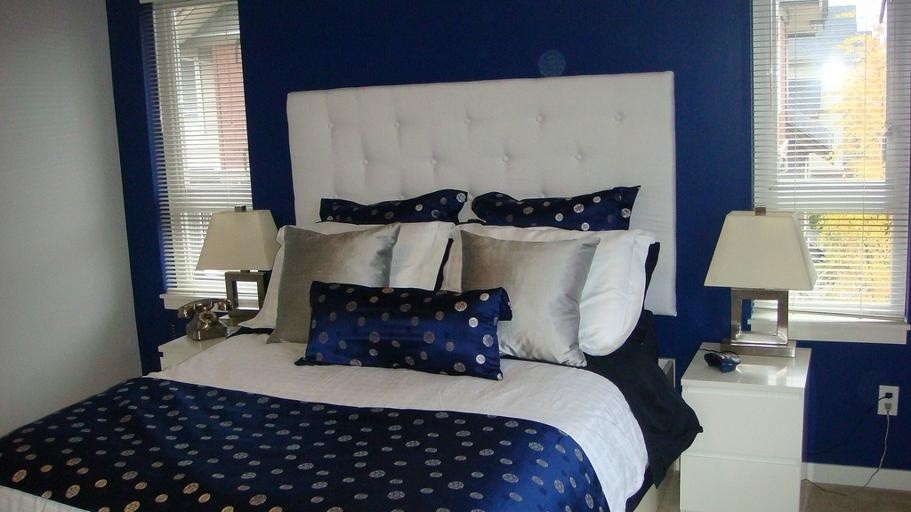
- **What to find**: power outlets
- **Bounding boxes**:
[877,385,900,417]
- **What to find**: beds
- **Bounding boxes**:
[0,72,701,512]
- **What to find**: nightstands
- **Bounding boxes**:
[678,342,813,512]
[159,323,227,371]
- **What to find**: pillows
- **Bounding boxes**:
[441,222,656,359]
[459,229,602,370]
[319,187,469,224]
[471,184,641,228]
[294,281,515,382]
[265,220,402,346]
[230,237,456,337]
[238,217,456,329]
[645,240,665,296]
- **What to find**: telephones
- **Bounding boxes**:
[178,298,233,341]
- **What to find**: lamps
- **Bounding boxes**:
[702,204,819,358]
[194,206,281,325]
